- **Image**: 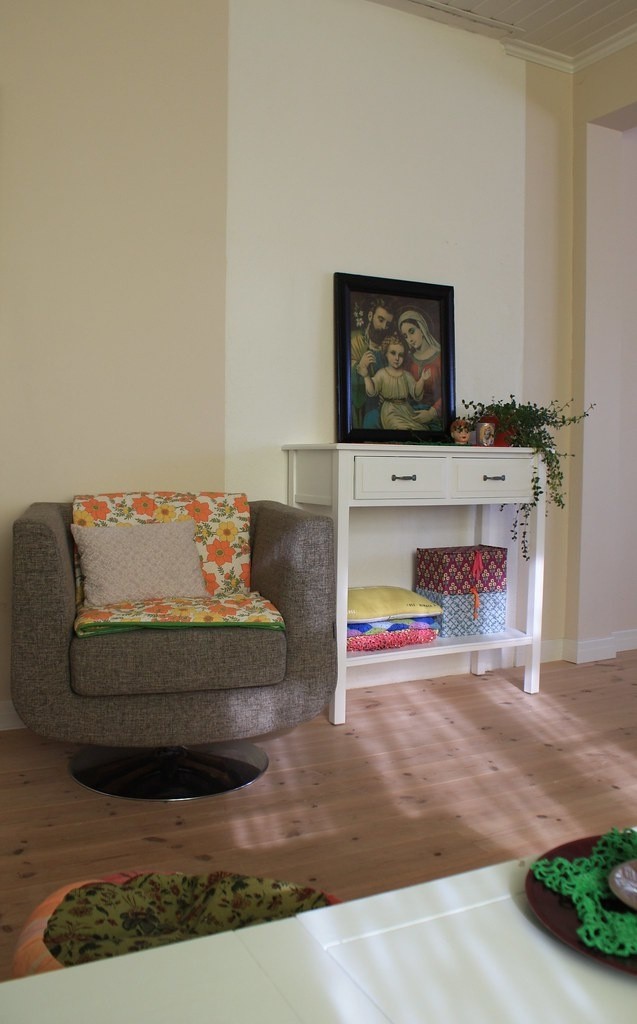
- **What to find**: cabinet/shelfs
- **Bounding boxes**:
[281,443,557,726]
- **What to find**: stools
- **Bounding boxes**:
[15,868,344,979]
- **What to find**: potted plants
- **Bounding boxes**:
[456,394,597,561]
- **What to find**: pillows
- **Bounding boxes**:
[70,519,209,604]
[347,587,444,624]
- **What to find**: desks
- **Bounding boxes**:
[0,826,636,1024]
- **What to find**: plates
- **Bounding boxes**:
[525,833,637,976]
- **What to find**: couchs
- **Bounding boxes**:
[12,490,337,803]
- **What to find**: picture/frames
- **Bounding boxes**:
[333,272,457,445]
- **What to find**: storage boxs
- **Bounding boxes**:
[417,544,507,638]
